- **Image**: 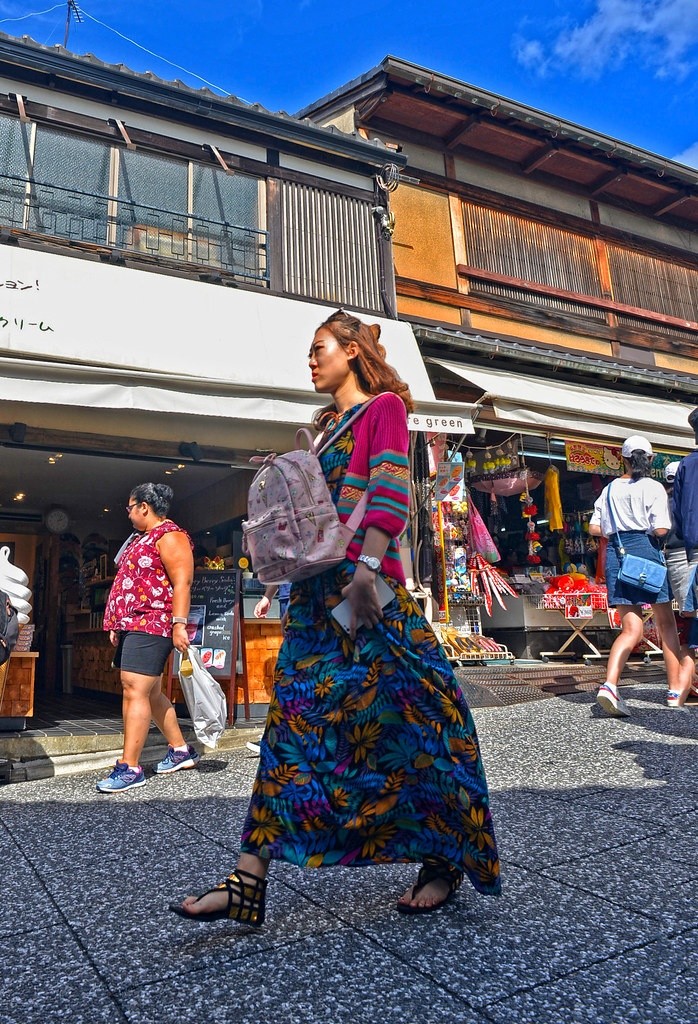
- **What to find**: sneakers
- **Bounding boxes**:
[151,744,200,774]
[96,760,146,793]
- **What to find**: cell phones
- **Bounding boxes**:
[331,573,397,634]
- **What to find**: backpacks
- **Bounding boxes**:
[242,392,408,585]
[0,589,19,666]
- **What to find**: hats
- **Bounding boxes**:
[621,435,654,458]
[665,461,681,483]
[688,407,698,431]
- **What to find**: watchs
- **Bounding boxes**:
[170,617,187,625]
[358,555,381,573]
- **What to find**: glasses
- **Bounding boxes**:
[126,503,150,514]
[329,306,349,318]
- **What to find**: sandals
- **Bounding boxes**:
[167,869,268,928]
[396,862,464,913]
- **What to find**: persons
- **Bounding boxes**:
[671,407,698,613]
[662,461,698,714]
[245,583,292,752]
[589,435,681,716]
[169,308,503,928]
[96,483,194,791]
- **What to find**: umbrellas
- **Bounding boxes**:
[472,477,541,496]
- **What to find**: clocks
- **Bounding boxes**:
[44,509,71,534]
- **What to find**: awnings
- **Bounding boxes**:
[426,356,698,450]
[0,244,482,438]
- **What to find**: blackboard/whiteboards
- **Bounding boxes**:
[167,569,248,678]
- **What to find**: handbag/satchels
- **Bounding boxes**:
[177,645,227,749]
[618,553,667,593]
[468,496,501,563]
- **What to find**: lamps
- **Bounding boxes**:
[179,441,204,462]
[8,422,26,441]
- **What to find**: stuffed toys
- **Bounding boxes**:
[563,562,586,573]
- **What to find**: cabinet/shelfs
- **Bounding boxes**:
[71,577,122,694]
[499,510,597,577]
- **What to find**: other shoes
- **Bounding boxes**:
[667,690,682,707]
[246,740,260,752]
[597,684,632,716]
